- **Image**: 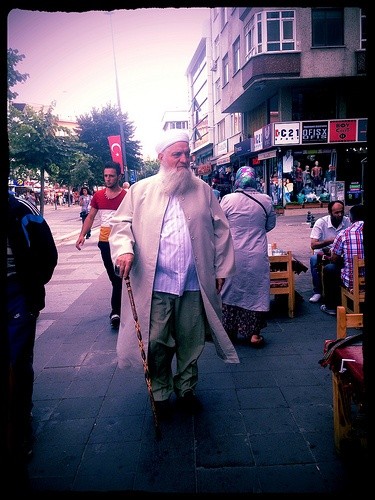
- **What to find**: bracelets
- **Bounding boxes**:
[322,255,327,260]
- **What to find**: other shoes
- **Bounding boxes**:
[154,393,204,425]
[110,314,120,324]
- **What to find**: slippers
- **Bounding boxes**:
[250,334,265,346]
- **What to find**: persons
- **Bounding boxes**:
[295,161,336,203]
[78,187,94,239]
[108,129,235,429]
[7,190,58,462]
[212,184,221,204]
[18,190,81,208]
[317,204,365,314]
[309,200,352,304]
[219,166,277,348]
[283,150,298,202]
[76,161,127,329]
[122,182,130,192]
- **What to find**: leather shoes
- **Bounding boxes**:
[85,234,90,239]
[309,292,321,303]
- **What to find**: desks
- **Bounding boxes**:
[318,339,363,454]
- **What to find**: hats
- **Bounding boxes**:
[149,129,192,152]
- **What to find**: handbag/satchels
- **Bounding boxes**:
[79,209,88,217]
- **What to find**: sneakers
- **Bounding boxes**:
[320,304,338,316]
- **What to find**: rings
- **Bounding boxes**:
[116,265,120,268]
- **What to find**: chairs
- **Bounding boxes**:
[341,255,365,329]
[268,251,294,318]
[336,306,363,340]
